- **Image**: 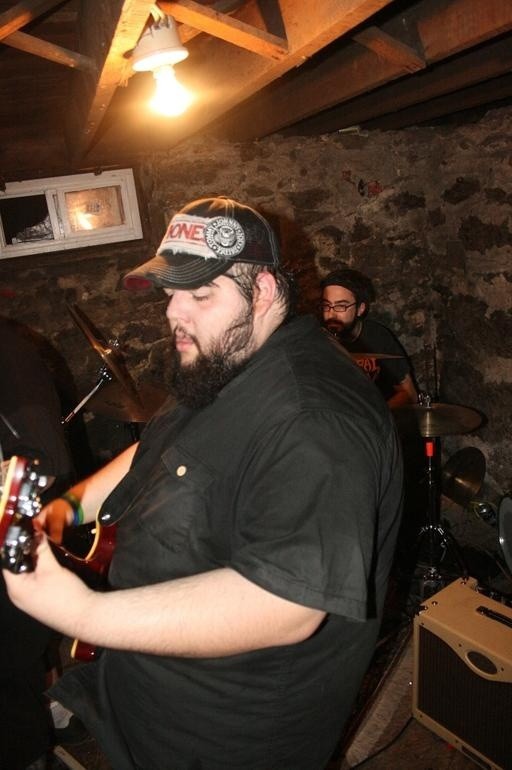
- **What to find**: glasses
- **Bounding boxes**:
[318,302,357,311]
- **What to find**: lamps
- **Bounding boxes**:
[128,9,199,121]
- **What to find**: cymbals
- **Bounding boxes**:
[345,354,406,360]
[87,380,162,425]
[405,400,484,439]
[438,447,486,505]
[69,303,136,398]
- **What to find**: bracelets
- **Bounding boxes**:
[61,492,83,528]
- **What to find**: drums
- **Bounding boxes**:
[496,493,511,575]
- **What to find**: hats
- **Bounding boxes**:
[121,195,282,289]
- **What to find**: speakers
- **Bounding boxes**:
[411,578,512,770]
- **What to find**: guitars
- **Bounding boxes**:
[1,453,118,665]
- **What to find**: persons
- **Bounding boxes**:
[0,194,407,770]
[318,268,419,411]
